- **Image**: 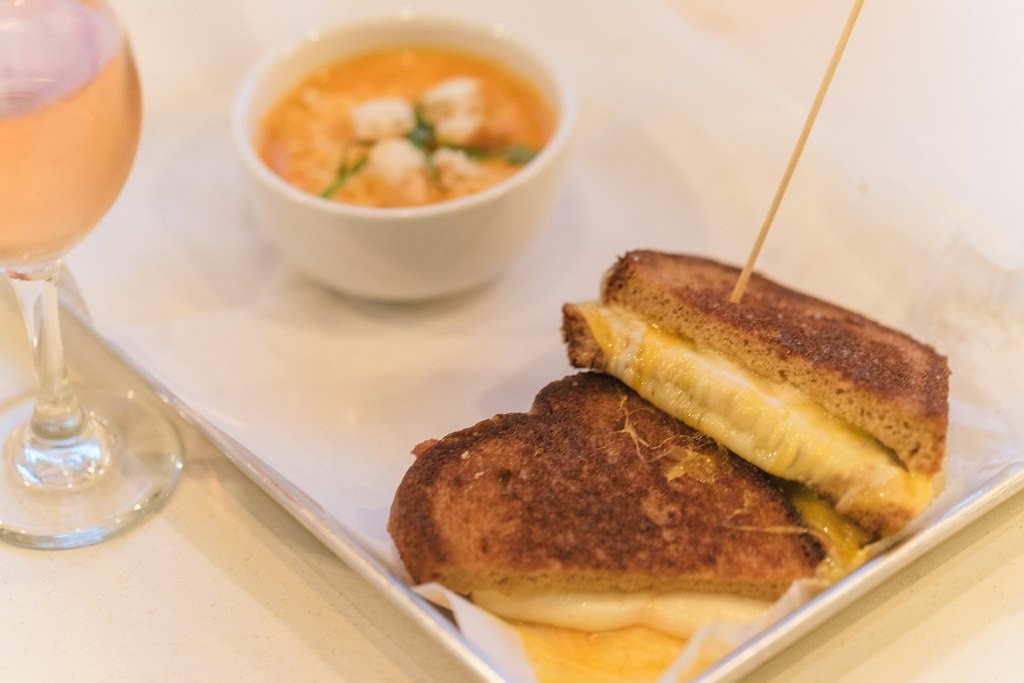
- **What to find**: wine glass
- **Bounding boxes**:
[0,0,185,551]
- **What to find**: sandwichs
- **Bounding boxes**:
[557,246,954,535]
[384,370,830,646]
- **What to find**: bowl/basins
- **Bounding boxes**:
[234,9,573,299]
